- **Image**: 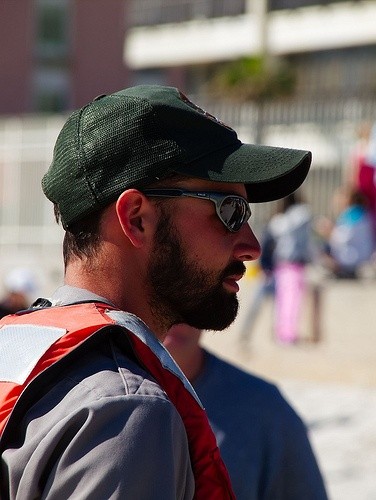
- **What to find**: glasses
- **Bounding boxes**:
[144,187,252,232]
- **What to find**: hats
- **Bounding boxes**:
[42,85,310,229]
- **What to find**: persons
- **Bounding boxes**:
[239,119,376,349]
[0,83,313,500]
[162,323,328,500]
